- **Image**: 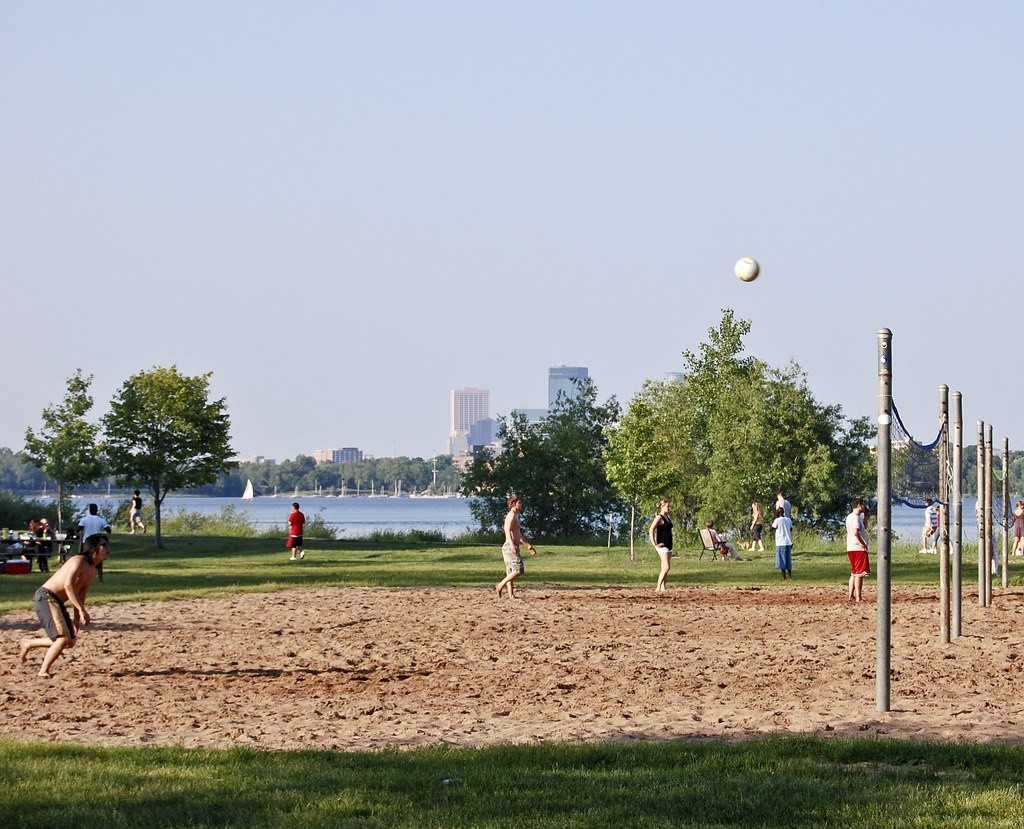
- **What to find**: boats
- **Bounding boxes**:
[366,478,466,501]
[271,486,281,498]
[291,486,302,499]
[326,486,337,498]
[312,478,324,498]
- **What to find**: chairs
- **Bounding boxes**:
[698,528,728,561]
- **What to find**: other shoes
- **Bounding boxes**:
[290,556,296,561]
[949,549,954,554]
[926,548,937,555]
[143,526,146,533]
[919,547,927,553]
[129,531,135,534]
[299,551,305,559]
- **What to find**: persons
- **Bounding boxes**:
[772,507,793,579]
[21,533,109,677]
[496,496,536,598]
[706,520,743,560]
[1012,501,1024,556]
[129,490,146,534]
[776,493,793,521]
[919,498,953,554]
[749,502,764,551]
[76,503,111,584]
[976,501,1000,576]
[0,520,53,572]
[649,499,673,592]
[846,500,871,602]
[288,503,305,560]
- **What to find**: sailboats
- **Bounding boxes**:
[338,480,354,498]
[241,479,256,500]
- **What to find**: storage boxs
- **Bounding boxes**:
[6,560,31,575]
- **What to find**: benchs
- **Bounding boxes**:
[1,553,70,572]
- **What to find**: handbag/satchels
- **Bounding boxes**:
[719,543,729,555]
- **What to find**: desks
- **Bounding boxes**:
[1,539,75,551]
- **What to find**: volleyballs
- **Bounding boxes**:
[734,256,761,283]
